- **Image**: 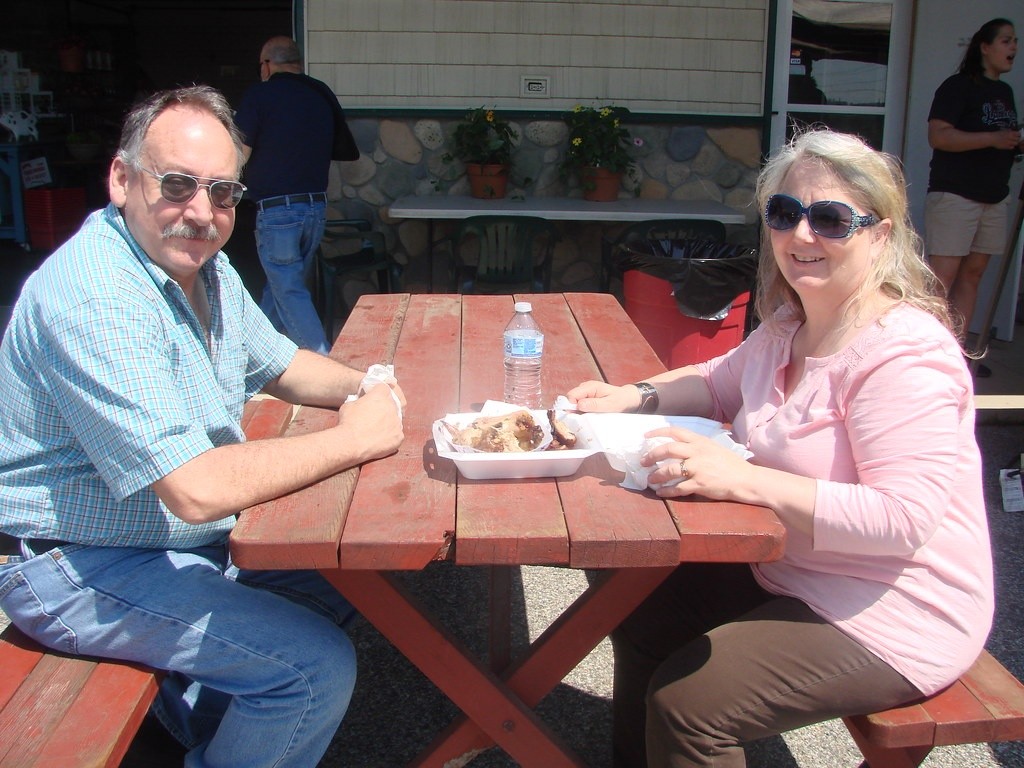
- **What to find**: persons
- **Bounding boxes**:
[559,127,998,768]
[923,17,1024,377]
[232,36,361,358]
[0,84,408,768]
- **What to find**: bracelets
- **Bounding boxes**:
[627,381,659,415]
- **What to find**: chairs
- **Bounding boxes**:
[600,218,726,293]
[451,213,557,295]
[315,219,403,344]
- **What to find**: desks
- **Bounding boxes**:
[230,291,933,768]
[388,196,745,294]
[0,137,71,249]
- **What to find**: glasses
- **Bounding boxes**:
[765,194,880,239]
[141,166,247,211]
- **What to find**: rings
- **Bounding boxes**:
[678,460,689,477]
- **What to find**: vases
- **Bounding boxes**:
[576,165,623,202]
[467,163,511,199]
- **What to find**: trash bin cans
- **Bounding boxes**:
[615,238,759,372]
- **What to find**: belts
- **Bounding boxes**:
[256,193,329,211]
[0,531,70,556]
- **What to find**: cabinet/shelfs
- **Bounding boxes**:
[41,0,139,144]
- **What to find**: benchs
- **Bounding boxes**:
[848,647,1024,750]
[0,399,294,768]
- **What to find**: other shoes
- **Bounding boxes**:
[967,360,991,379]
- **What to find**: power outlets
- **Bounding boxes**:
[520,75,550,99]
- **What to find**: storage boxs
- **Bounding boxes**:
[24,187,85,250]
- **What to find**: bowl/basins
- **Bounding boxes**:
[433,412,752,480]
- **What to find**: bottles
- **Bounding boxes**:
[504,303,545,409]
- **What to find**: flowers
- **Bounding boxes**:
[417,104,537,201]
[557,96,645,200]
